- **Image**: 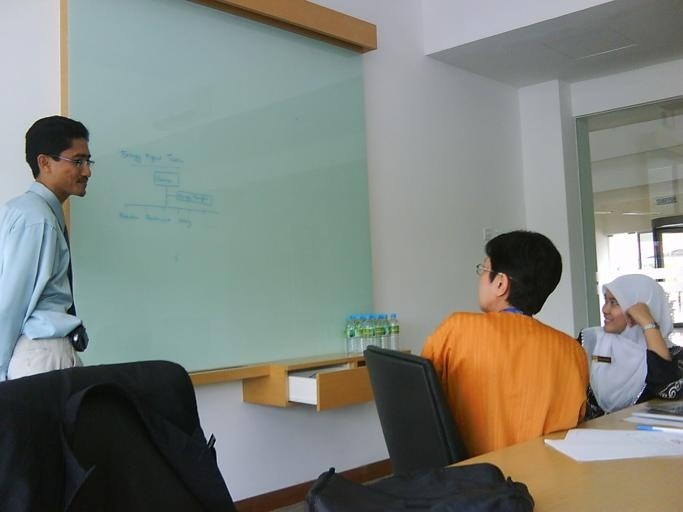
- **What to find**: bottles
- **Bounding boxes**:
[344,313,399,353]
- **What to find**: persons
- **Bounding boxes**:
[571,272,683,419]
[420,229,589,463]
[0,115,93,389]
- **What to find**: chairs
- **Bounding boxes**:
[364,345,472,475]
[0,361,238,512]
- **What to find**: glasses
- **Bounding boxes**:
[44,151,96,169]
[476,264,515,281]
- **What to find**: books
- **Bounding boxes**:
[630,403,682,421]
[623,413,682,428]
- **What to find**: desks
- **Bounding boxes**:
[444,396,683,512]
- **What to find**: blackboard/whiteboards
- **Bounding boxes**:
[61,0,378,383]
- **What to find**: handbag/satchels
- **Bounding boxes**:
[70,324,89,352]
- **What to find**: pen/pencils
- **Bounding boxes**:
[636,425,683,434]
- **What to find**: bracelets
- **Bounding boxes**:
[640,322,659,334]
